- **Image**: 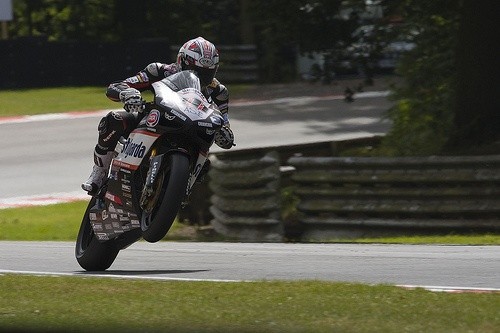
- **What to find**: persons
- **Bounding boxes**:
[81,37,235,193]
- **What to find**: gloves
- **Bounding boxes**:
[118,87,143,113]
[212,124,235,149]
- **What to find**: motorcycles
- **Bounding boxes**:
[75,69,236,271]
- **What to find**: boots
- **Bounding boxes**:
[180,153,211,209]
[81,143,116,192]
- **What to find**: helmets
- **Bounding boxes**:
[176,37,220,87]
